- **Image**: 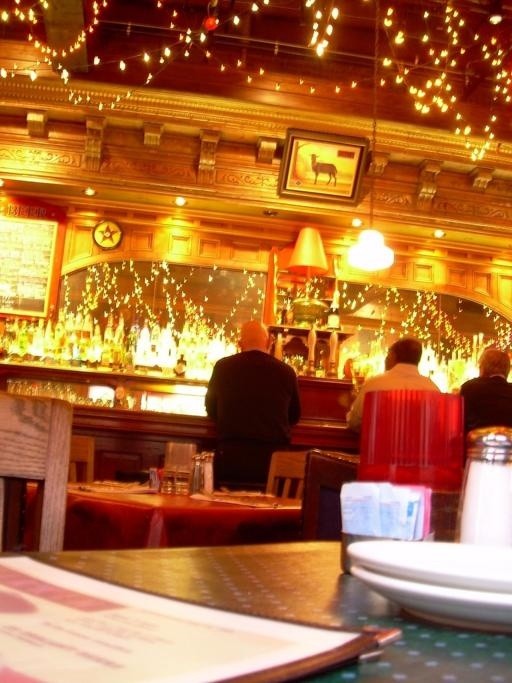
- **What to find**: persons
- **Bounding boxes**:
[204,321,302,494]
[344,338,443,431]
[457,347,512,436]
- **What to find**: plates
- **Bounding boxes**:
[343,538,511,631]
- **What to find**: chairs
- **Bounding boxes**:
[269,449,461,542]
[1,392,96,551]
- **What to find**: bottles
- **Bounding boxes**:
[0,311,189,378]
[302,358,339,380]
[457,428,512,542]
[189,449,217,494]
[326,289,341,330]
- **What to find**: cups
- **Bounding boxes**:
[172,470,190,494]
[159,467,173,491]
[4,375,113,408]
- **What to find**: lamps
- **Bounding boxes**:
[286,227,331,323]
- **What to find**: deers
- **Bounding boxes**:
[311,153,338,187]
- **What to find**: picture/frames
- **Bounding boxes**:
[274,126,372,206]
